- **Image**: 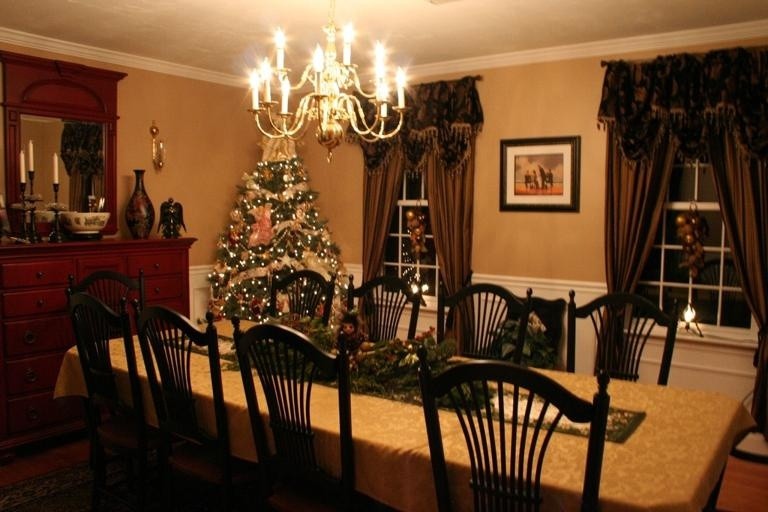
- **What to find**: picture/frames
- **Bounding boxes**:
[498,138,581,214]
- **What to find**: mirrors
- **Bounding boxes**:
[17,111,107,230]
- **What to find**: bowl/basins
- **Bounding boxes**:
[61,212,111,234]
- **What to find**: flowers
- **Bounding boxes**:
[226,312,552,409]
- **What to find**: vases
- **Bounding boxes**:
[125,170,155,239]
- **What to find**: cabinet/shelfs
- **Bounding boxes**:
[0,232,197,457]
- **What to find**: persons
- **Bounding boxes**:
[337,316,359,356]
[525,166,554,189]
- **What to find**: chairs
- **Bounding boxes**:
[566,290,678,388]
[348,275,421,340]
[232,313,355,507]
[266,272,335,324]
[437,280,531,367]
[419,366,611,512]
[131,299,227,491]
[63,292,143,470]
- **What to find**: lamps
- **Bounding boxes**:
[680,302,702,327]
[244,23,407,148]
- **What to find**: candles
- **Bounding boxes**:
[19,139,59,184]
